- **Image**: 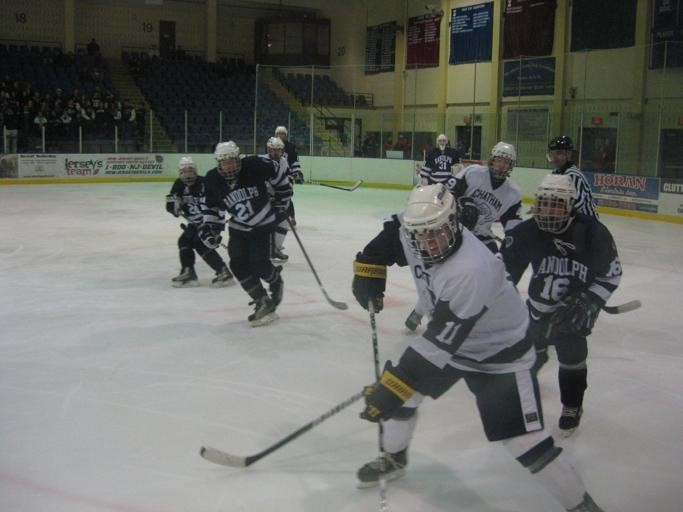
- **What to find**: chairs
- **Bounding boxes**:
[0,44,376,157]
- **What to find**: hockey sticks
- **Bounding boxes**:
[300,178,362,191]
[200,385,381,467]
[476,224,641,314]
[286,215,347,310]
[368,297,387,511]
[178,211,283,274]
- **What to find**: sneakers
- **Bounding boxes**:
[559,405,582,429]
[359,451,407,482]
[248,276,281,320]
[272,249,288,259]
[172,268,235,282]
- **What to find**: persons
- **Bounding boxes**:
[0,37,146,141]
[258,136,293,260]
[351,182,605,511]
[498,172,623,429]
[274,121,305,183]
[419,132,462,186]
[452,140,526,250]
[546,133,600,222]
[198,140,295,322]
[351,130,473,160]
[175,44,186,61]
[165,164,233,284]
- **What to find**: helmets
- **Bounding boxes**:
[534,174,578,234]
[178,157,196,185]
[215,140,242,179]
[546,135,573,162]
[490,141,516,177]
[404,183,458,263]
[436,134,448,151]
[267,126,288,150]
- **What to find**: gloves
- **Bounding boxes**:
[457,195,478,230]
[352,252,386,312]
[198,223,222,248]
[549,292,599,336]
[361,360,418,422]
